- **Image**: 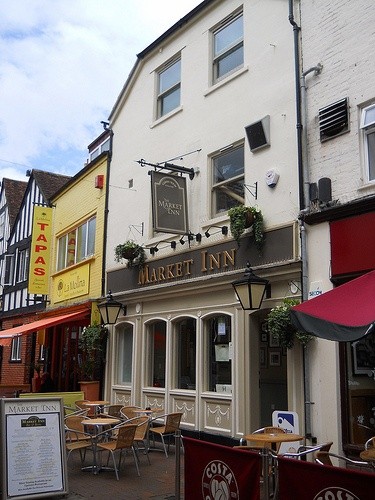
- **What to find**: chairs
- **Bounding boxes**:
[64,396,184,481]
[232,424,375,474]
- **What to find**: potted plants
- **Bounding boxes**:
[77,361,100,401]
[227,204,266,247]
[113,240,145,267]
[264,295,316,351]
[78,323,109,354]
[29,362,42,392]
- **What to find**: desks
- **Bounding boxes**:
[130,407,165,455]
[241,432,304,453]
[360,448,375,461]
[78,417,121,475]
[83,398,112,442]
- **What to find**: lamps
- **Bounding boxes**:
[139,246,154,254]
[152,240,176,251]
[203,225,228,239]
[179,232,202,245]
[96,289,128,327]
[230,261,276,312]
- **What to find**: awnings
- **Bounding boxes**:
[291,269,375,342]
[0,307,91,348]
